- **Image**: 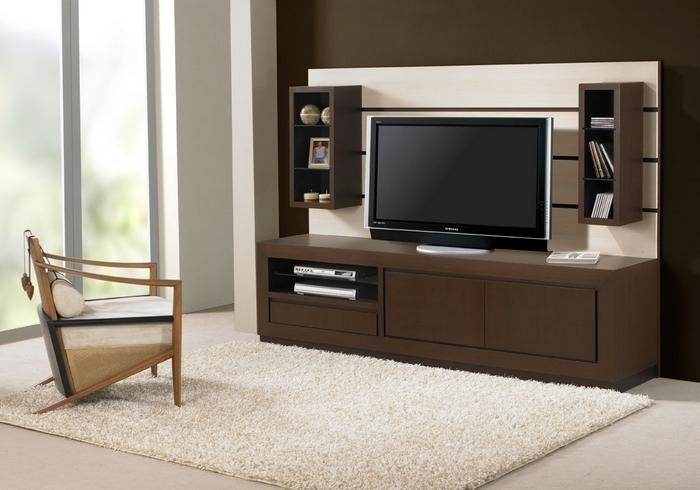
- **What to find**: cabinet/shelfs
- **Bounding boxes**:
[255,233,659,393]
[578,81,644,226]
[290,85,363,211]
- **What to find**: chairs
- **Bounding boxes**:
[21,230,182,417]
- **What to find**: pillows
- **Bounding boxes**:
[47,270,85,318]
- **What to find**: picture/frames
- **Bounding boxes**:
[308,137,330,169]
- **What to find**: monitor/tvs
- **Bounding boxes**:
[362,114,555,255]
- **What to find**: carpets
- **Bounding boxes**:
[0,338,655,490]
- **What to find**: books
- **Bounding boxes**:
[545,250,600,265]
[590,116,615,130]
[591,193,614,220]
[588,140,614,179]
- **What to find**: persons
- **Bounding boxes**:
[313,142,328,163]
